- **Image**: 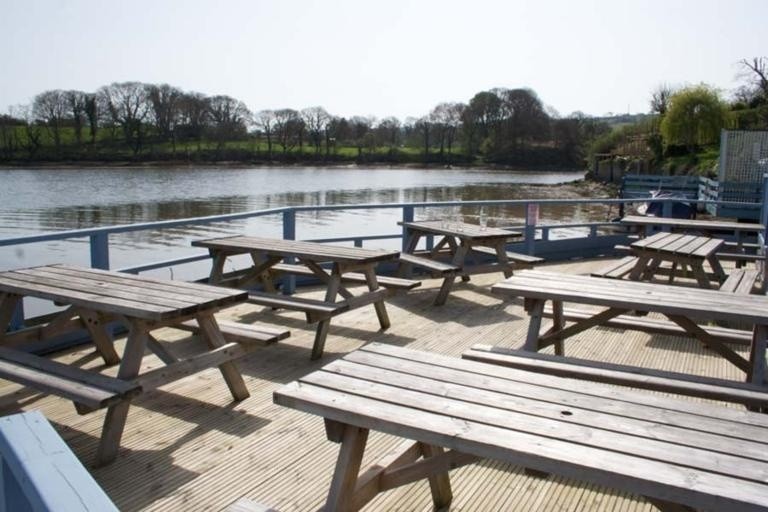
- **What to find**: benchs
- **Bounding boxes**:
[379,245,546,305]
[1,342,143,470]
[538,304,754,356]
[206,262,421,359]
[590,235,766,295]
[462,341,767,412]
[72,307,290,401]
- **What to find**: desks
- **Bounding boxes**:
[620,214,766,288]
[191,234,401,360]
[0,261,251,469]
[273,340,767,512]
[395,219,522,306]
[492,268,767,386]
